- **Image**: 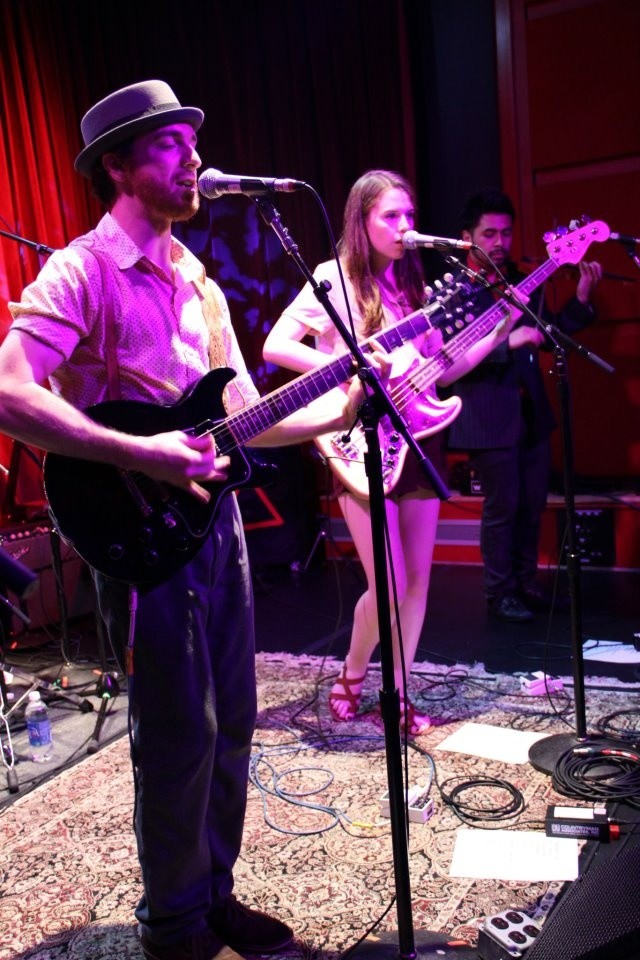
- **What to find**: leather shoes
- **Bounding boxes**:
[489,593,532,620]
[521,582,571,610]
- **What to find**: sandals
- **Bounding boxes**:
[399,696,435,735]
[329,659,368,723]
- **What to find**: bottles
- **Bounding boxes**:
[24,690,54,763]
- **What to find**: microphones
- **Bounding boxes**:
[198,167,305,200]
[606,231,640,242]
[401,230,480,251]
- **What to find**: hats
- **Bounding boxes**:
[74,79,205,180]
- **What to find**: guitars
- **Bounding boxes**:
[315,215,618,498]
[43,276,480,578]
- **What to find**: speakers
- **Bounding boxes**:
[520,840,640,960]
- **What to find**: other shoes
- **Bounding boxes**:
[206,892,293,955]
[142,925,247,960]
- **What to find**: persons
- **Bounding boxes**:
[428,191,602,623]
[0,79,391,960]
[262,169,530,736]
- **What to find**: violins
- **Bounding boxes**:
[499,250,640,284]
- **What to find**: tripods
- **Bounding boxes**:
[41,611,128,756]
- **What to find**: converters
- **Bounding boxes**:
[544,804,611,842]
